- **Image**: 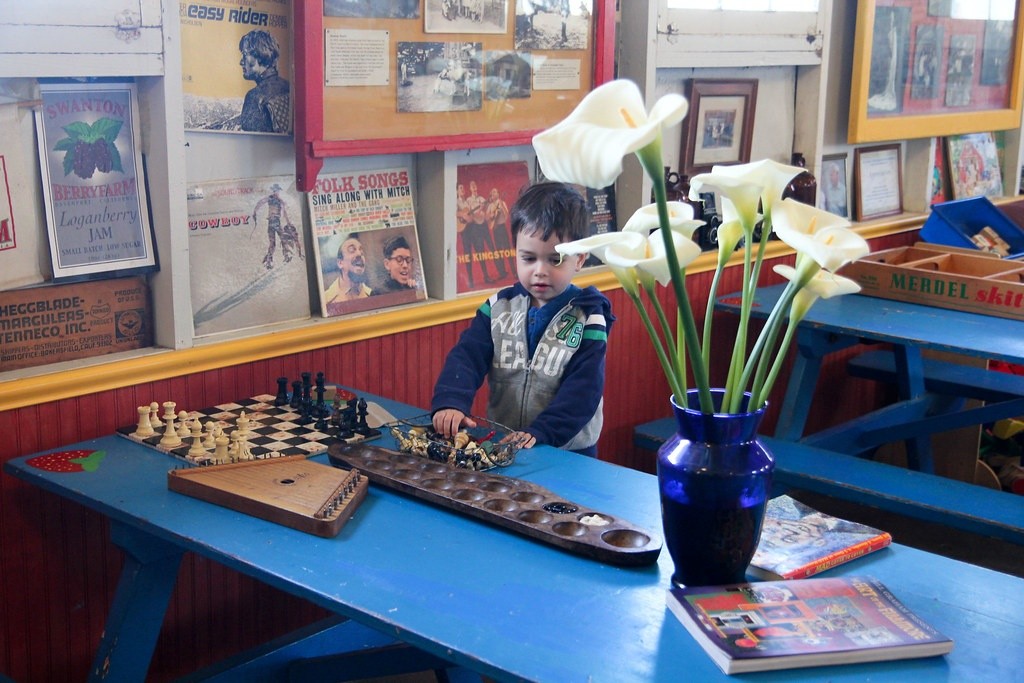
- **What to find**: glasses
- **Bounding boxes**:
[388,256,414,263]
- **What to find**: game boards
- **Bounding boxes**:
[115,394,384,468]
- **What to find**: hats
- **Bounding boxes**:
[327,232,358,260]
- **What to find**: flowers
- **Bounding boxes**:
[530,78,871,413]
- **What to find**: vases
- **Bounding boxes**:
[655,386,776,588]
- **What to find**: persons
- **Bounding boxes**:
[823,162,846,218]
[233,29,292,133]
[457,179,518,288]
[251,184,294,268]
[428,179,619,463]
[323,235,419,304]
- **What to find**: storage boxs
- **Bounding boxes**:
[844,245,1024,321]
[918,195,1024,259]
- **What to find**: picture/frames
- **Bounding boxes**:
[846,0,1024,146]
[679,78,759,180]
[290,0,617,192]
[820,153,848,217]
[854,142,904,221]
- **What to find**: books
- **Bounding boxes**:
[663,576,953,677]
[745,494,893,581]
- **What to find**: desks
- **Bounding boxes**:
[714,280,1024,456]
[3,379,1024,683]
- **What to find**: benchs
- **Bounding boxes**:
[633,417,1023,548]
[846,348,1024,473]
